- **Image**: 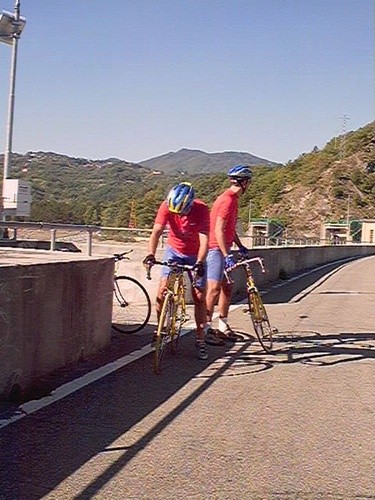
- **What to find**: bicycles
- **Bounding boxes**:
[111,249,151,335]
[144,260,199,372]
[222,251,273,349]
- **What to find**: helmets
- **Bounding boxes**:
[227,165,253,177]
[166,182,194,214]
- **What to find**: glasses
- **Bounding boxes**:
[248,179,252,183]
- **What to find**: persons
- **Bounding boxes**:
[142,182,210,360]
[206,164,253,346]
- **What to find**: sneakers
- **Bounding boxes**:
[218,328,244,342]
[203,328,225,346]
[193,338,208,360]
[150,330,162,347]
